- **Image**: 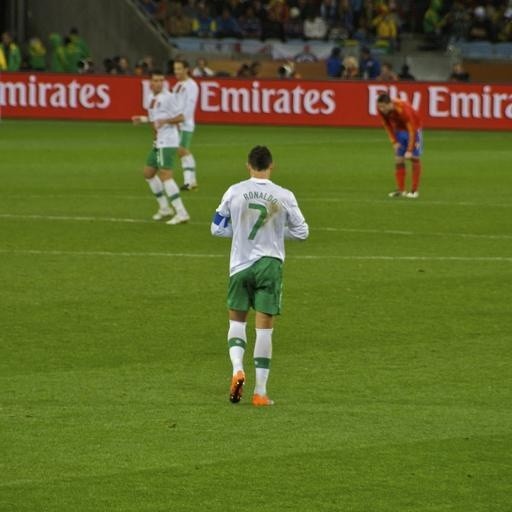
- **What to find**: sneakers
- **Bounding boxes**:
[165,214,190,226]
[252,393,276,406]
[229,370,244,403]
[388,190,418,200]
[152,208,174,219]
[179,183,199,191]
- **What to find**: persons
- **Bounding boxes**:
[423,1,511,82]
[0,26,96,73]
[171,60,200,189]
[326,47,415,83]
[376,94,422,199]
[210,146,310,406]
[139,1,404,54]
[108,55,215,77]
[131,69,190,225]
[237,45,317,79]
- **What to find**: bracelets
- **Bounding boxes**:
[140,115,148,124]
